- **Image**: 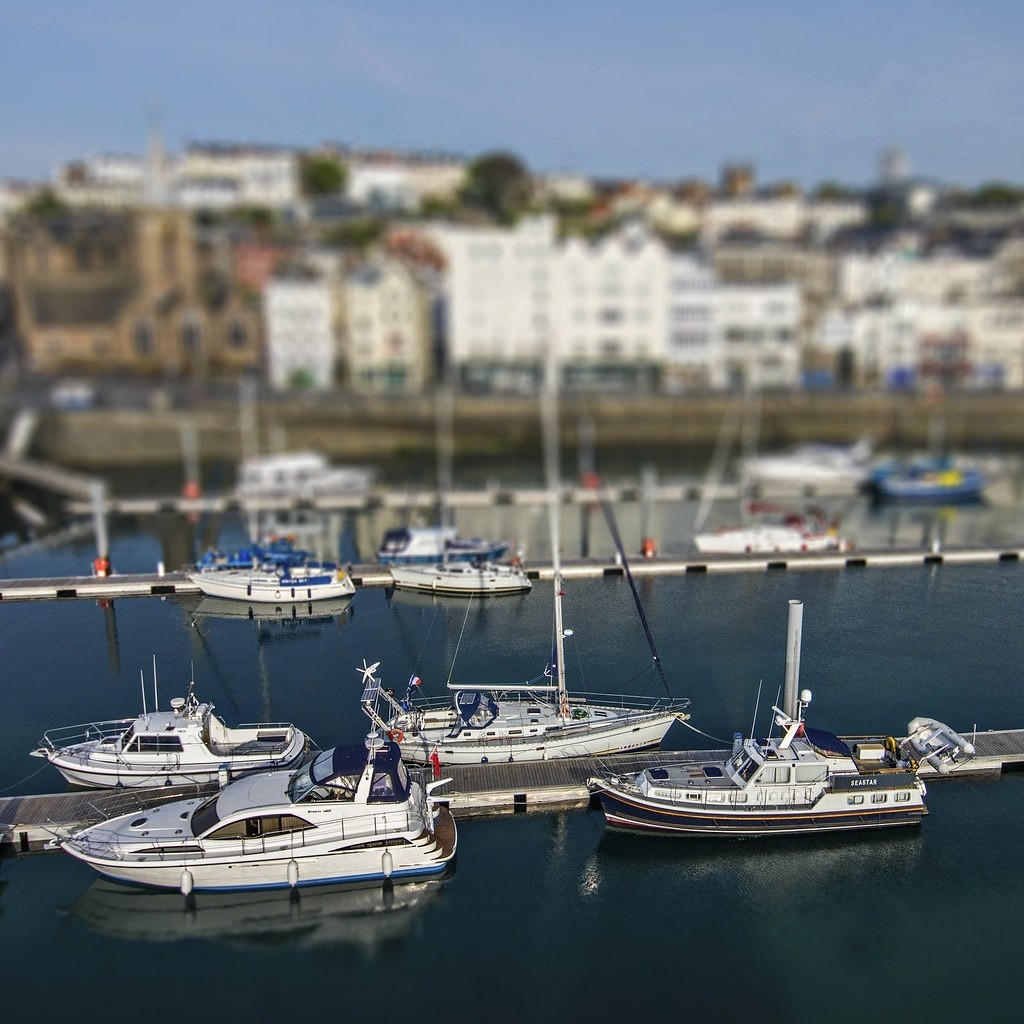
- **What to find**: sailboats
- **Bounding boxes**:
[358,360,694,770]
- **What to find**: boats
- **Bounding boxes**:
[693,500,844,555]
[49,714,458,896]
[27,653,319,788]
[584,677,975,836]
[387,559,533,597]
[732,435,872,493]
[186,533,357,602]
[234,451,373,515]
[861,448,993,504]
[375,525,506,566]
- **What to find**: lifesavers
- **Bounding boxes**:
[910,758,917,773]
[886,737,896,753]
[389,729,403,744]
